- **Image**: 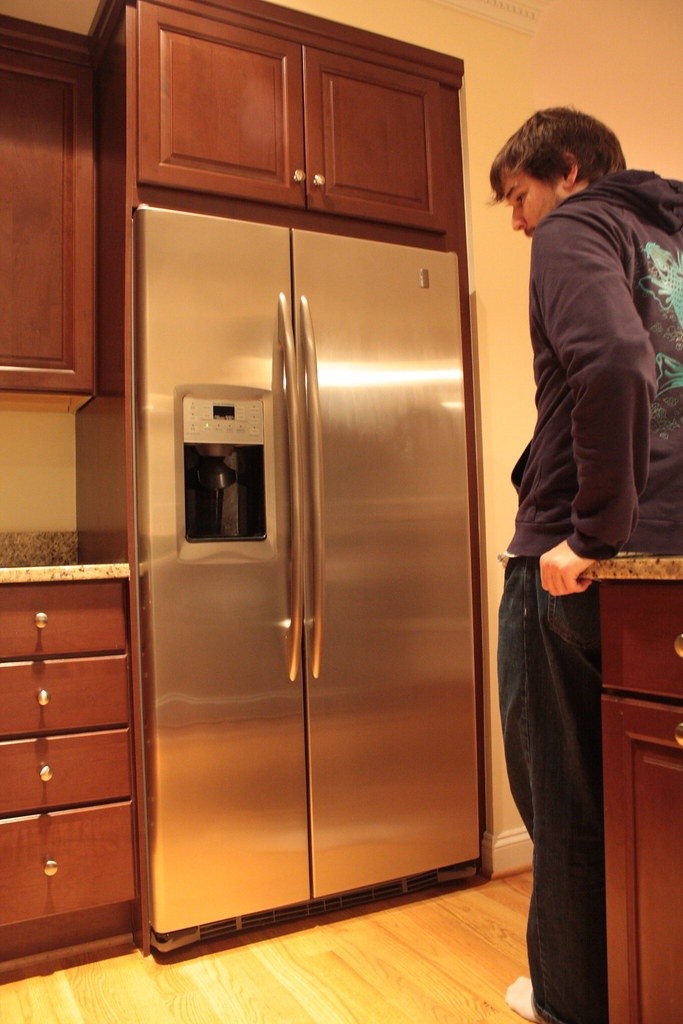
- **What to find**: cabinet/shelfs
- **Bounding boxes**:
[0,13,96,415]
[0,578,136,985]
[97,0,465,235]
[577,581,683,1024]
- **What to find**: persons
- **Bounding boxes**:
[490,105,683,1024]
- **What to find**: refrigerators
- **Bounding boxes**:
[131,206,480,954]
